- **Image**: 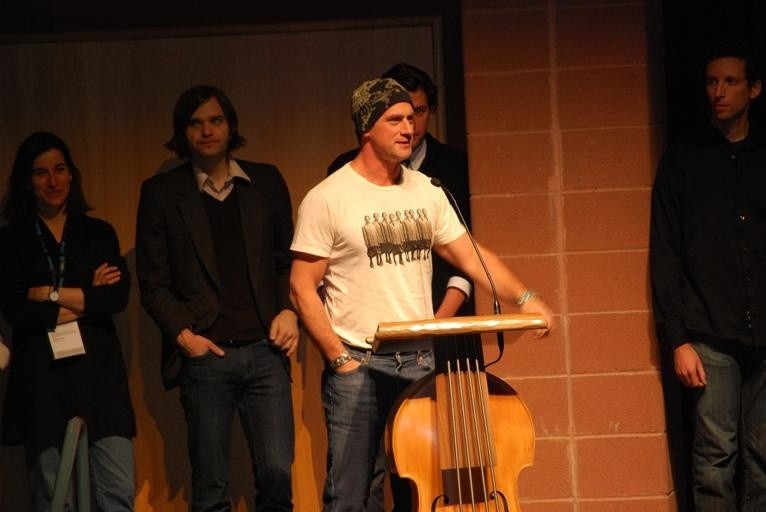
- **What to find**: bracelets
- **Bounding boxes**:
[331,350,351,370]
[516,288,535,308]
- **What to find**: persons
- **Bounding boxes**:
[649,40,766,512]
[327,63,487,511]
[288,78,555,511]
[0,130,137,512]
[135,85,301,512]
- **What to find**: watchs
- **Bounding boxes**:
[49,286,60,304]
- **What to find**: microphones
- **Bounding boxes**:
[430,177,501,316]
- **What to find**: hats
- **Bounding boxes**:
[350,75,416,146]
